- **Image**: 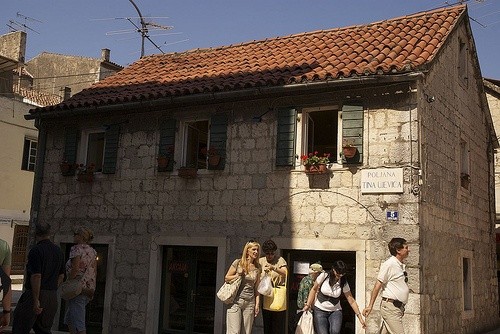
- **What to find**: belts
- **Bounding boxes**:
[382,297,405,307]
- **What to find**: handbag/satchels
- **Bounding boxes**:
[264,268,288,313]
[216,259,242,305]
[58,277,83,301]
[294,310,316,334]
[257,272,274,298]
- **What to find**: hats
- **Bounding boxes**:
[309,263,326,273]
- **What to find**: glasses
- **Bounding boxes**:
[335,269,346,275]
[403,271,408,283]
[74,233,79,237]
[248,239,258,244]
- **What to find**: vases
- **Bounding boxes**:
[177,168,198,177]
[303,164,327,174]
[208,154,219,167]
[78,174,96,183]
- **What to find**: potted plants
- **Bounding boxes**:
[461,172,471,189]
[155,152,169,167]
[339,143,358,159]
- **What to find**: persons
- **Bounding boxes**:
[0,240,12,329]
[63,226,97,334]
[254,240,288,334]
[361,237,410,334]
[297,263,325,324]
[226,239,262,334]
[302,260,367,334]
[11,223,65,334]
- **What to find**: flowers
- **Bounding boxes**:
[76,163,96,176]
[200,144,217,155]
[300,151,330,168]
